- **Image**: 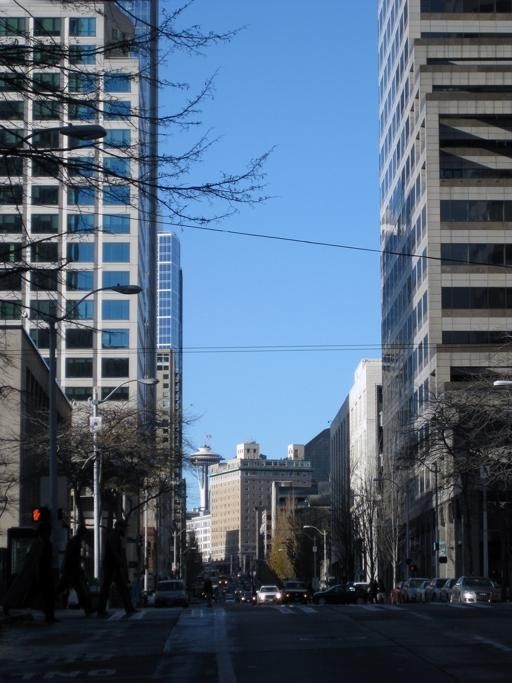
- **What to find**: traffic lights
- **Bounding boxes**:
[31,505,42,523]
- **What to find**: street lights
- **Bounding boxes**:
[302,525,328,580]
[374,455,440,577]
[46,284,159,581]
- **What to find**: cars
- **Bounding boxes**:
[389,574,505,605]
[283,579,309,606]
[240,591,257,605]
[154,579,190,609]
[313,583,371,605]
[256,584,283,606]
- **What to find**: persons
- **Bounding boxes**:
[38,522,97,615]
[93,518,142,619]
[24,522,65,623]
[203,577,214,608]
[368,578,380,603]
